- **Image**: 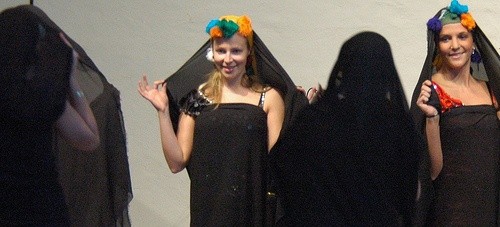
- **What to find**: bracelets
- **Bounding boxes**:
[73,90,84,97]
[426,113,439,118]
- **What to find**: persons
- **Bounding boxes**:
[0,5,133,227]
[411,0,500,227]
[270,32,421,227]
[138,15,322,227]
